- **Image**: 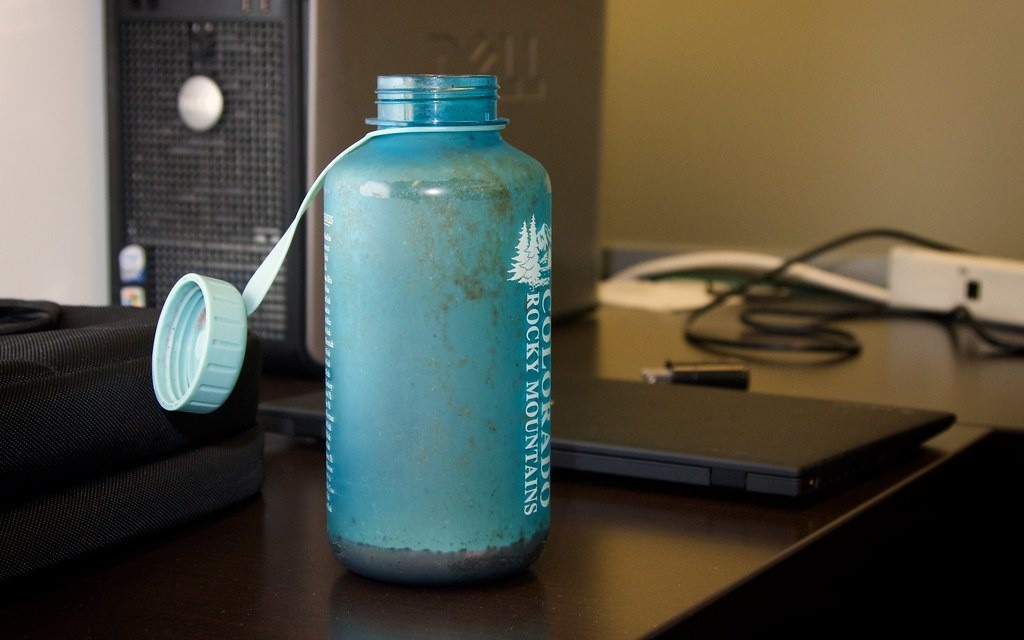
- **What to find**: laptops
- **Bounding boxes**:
[256,376,958,496]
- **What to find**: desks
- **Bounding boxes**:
[0,296,1024,640]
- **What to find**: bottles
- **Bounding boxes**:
[151,69,552,584]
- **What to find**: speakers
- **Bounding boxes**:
[105,0,608,386]
